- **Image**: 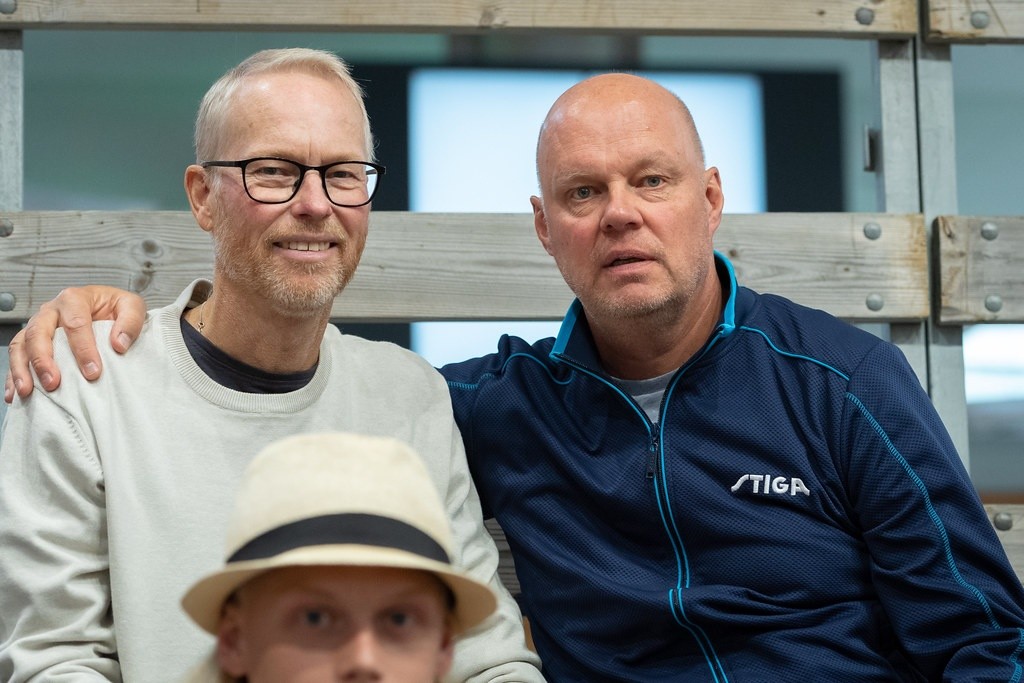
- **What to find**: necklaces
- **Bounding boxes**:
[198,300,208,333]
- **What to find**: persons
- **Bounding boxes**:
[0,49,1024,683]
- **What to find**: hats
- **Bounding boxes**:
[179,431,498,636]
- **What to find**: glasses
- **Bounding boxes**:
[200,154,387,207]
